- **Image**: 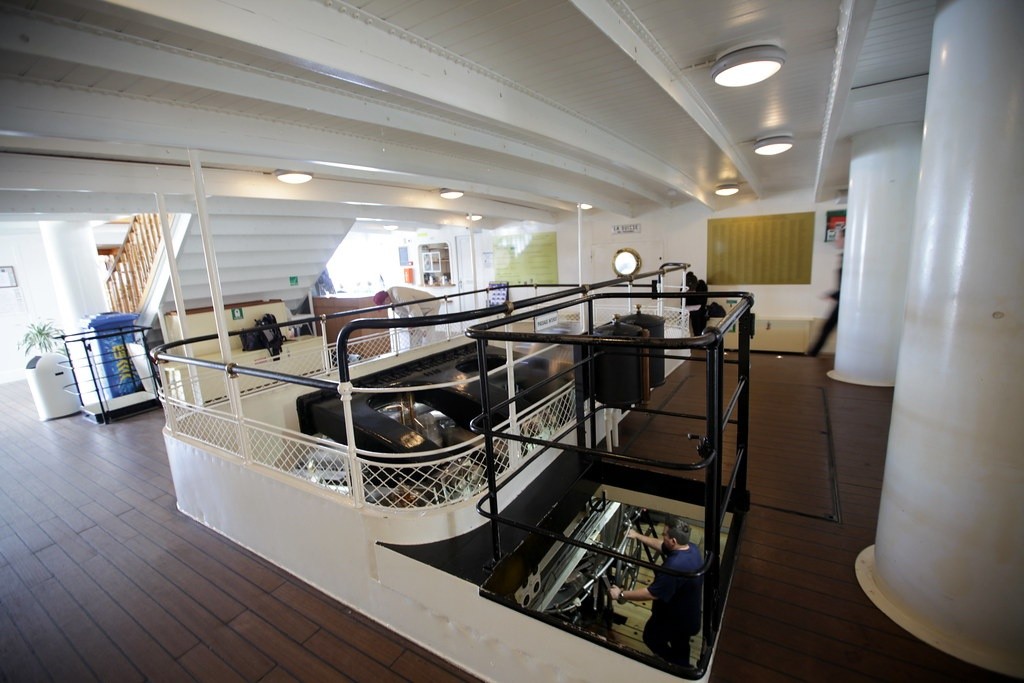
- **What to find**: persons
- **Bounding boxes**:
[679,270,709,339]
[373,284,442,349]
[608,518,701,669]
[803,226,848,356]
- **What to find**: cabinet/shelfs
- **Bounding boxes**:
[310,293,392,360]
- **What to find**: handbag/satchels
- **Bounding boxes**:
[240,330,267,350]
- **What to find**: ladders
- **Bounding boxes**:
[635,512,666,575]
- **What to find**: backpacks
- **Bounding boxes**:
[256,313,283,346]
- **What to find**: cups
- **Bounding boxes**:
[442,276,447,284]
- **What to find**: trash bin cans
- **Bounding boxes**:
[80,311,145,398]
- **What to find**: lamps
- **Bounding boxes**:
[712,45,787,87]
[754,136,794,156]
[714,184,739,196]
[441,188,464,198]
[274,169,313,184]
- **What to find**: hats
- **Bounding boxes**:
[373,291,387,311]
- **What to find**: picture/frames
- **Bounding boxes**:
[0,265,18,287]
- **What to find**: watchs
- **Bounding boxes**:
[619,590,624,598]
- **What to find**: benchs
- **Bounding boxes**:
[159,300,328,405]
[692,315,814,352]
